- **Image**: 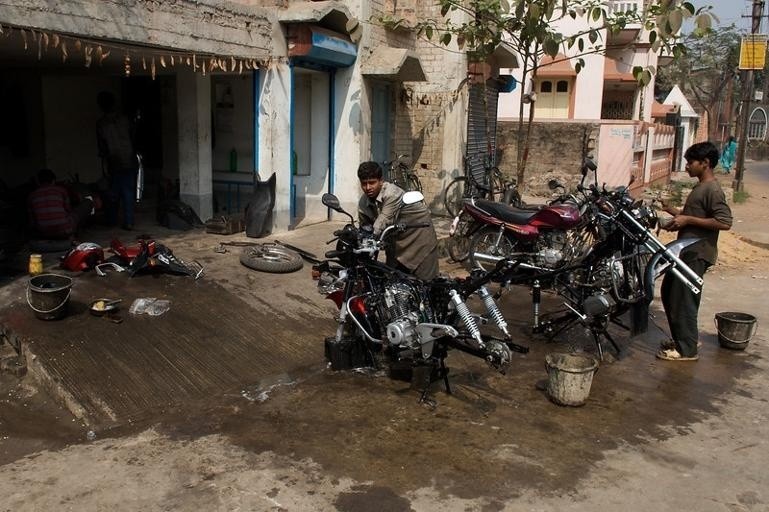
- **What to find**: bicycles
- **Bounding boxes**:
[444,151,518,220]
[380,154,423,193]
[447,207,469,263]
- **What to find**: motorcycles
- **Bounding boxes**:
[312,192,530,403]
[463,160,707,362]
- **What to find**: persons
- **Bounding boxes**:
[32,169,78,237]
[720,135,737,173]
[654,140,733,361]
[356,161,440,282]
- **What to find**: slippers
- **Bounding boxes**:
[660,338,675,348]
[657,347,699,362]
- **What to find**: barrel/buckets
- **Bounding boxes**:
[25,273,74,321]
[713,310,759,351]
[543,352,600,408]
